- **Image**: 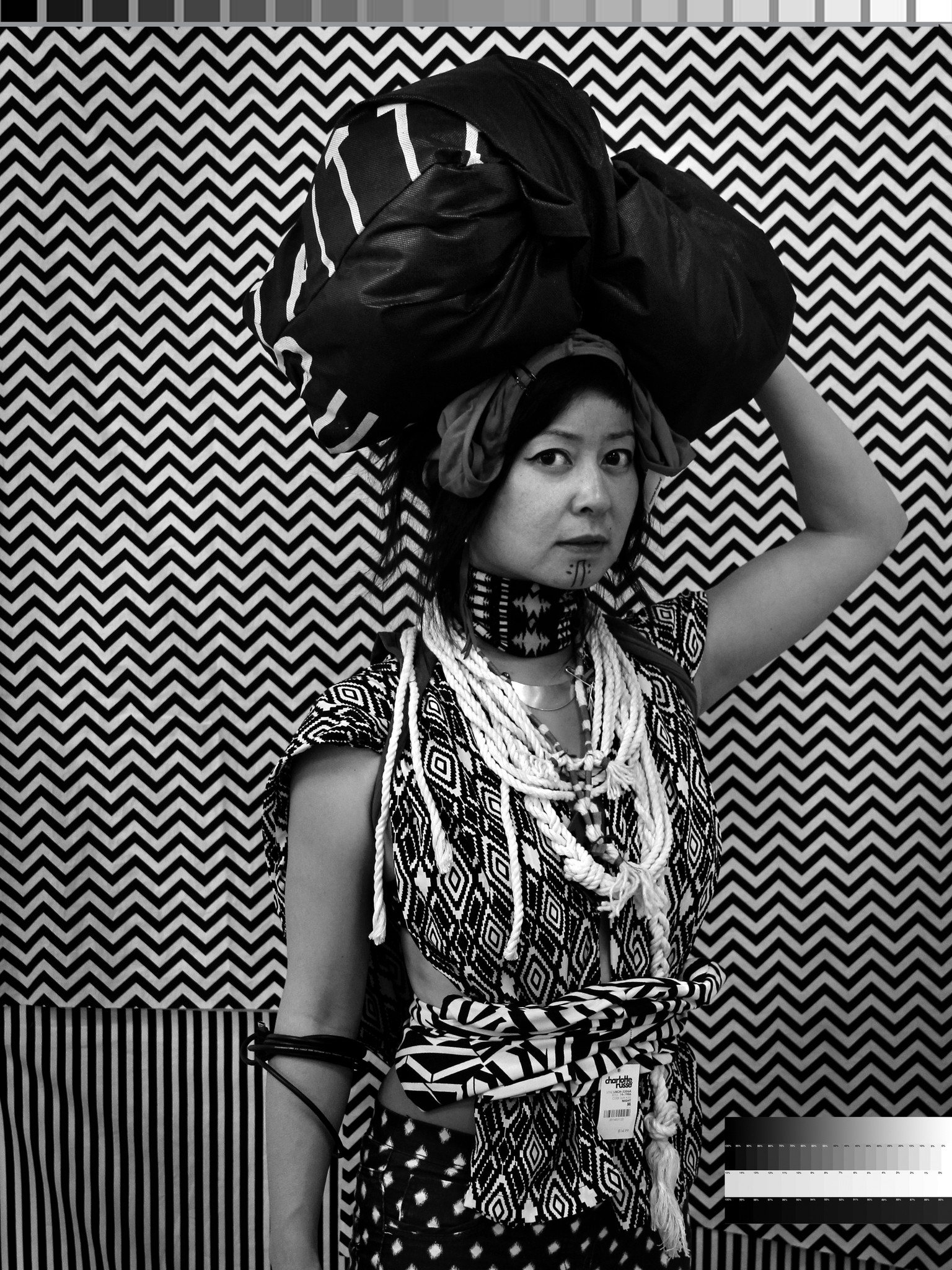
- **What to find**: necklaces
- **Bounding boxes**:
[370,580,695,1260]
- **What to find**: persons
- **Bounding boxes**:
[244,324,912,1270]
